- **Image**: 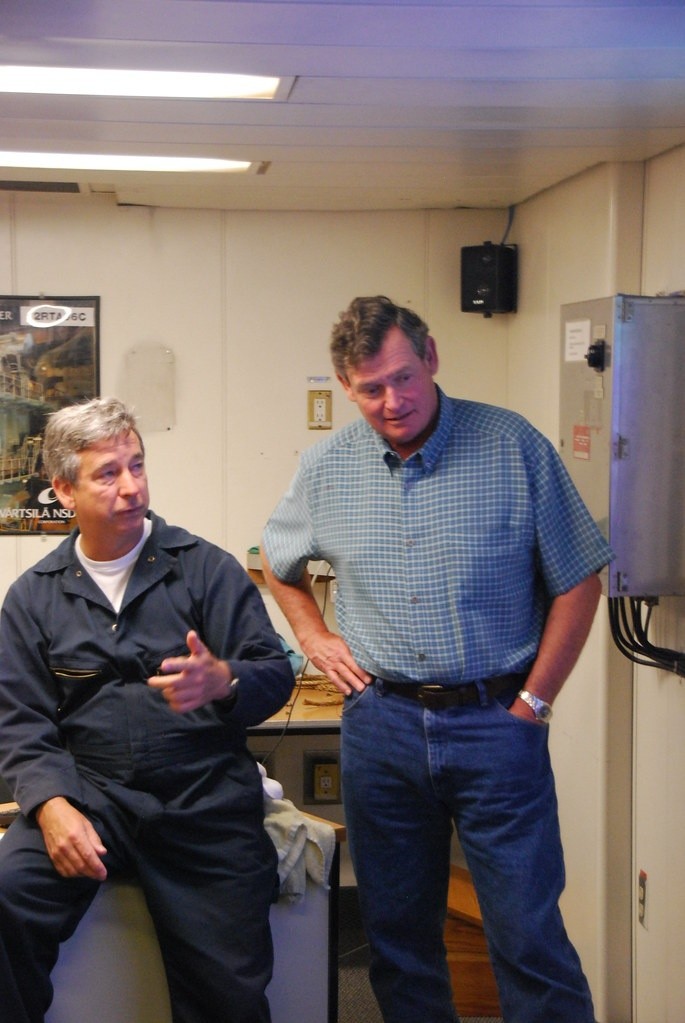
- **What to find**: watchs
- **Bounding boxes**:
[222,666,240,700]
[516,689,554,723]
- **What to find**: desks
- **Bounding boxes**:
[0,808,346,1023]
[247,674,342,738]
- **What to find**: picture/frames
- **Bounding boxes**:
[0,295,101,535]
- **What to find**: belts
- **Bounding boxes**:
[373,672,526,710]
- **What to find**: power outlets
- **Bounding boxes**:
[303,748,343,805]
[307,390,333,430]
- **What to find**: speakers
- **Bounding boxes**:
[460,244,516,313]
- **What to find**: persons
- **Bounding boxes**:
[258,294,616,1023]
[1,397,295,1023]
